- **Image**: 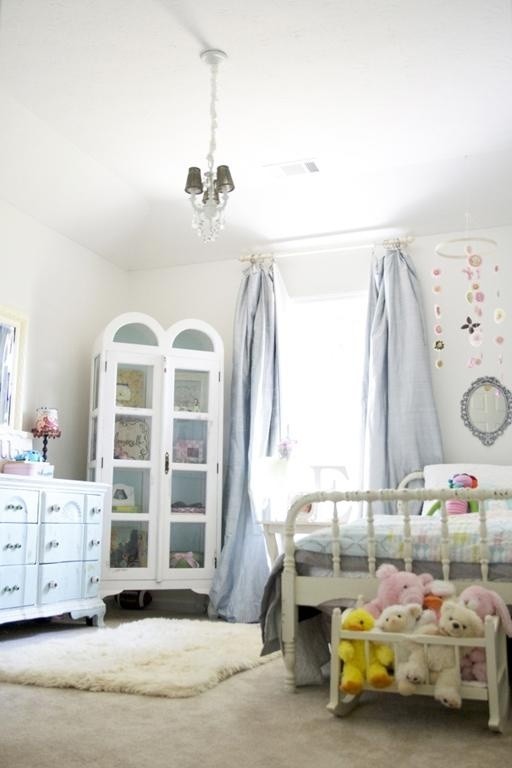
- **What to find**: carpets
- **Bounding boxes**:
[0,618,281,697]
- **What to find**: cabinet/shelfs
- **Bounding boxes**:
[88,311,225,618]
[0,474,111,628]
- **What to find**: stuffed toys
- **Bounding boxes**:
[337,560,512,712]
[427,472,479,515]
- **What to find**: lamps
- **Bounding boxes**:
[184,50,235,244]
[31,405,62,461]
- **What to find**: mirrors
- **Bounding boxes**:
[460,376,512,447]
[0,306,30,432]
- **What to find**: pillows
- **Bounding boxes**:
[421,464,512,517]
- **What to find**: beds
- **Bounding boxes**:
[260,472,512,694]
